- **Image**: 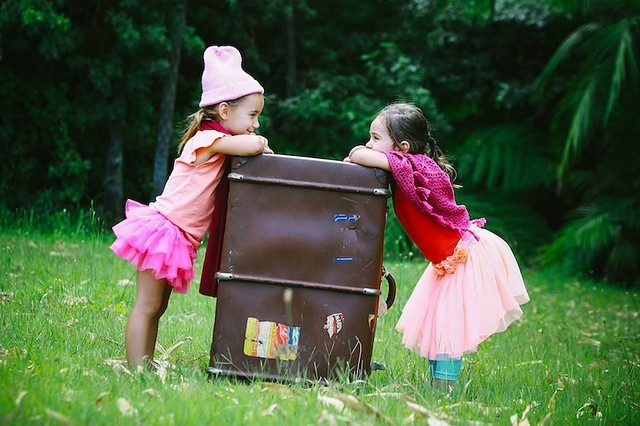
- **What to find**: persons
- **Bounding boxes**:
[344,102,531,392]
[109,44,273,381]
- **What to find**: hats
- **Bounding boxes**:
[200,46,264,107]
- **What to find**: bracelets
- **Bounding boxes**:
[348,146,366,156]
